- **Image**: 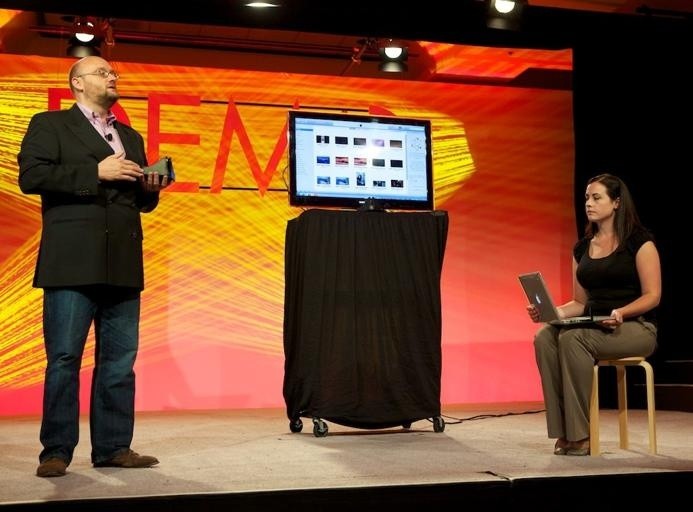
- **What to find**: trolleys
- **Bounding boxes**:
[278,205,452,442]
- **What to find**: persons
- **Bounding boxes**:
[17,55,175,476]
[527,174,663,456]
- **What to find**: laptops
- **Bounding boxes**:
[519,271,615,325]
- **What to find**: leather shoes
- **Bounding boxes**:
[554,438,590,455]
[37,457,66,476]
[94,448,159,468]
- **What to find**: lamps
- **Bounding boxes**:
[483,0,523,35]
[58,18,99,62]
[373,40,413,76]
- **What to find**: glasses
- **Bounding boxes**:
[78,68,117,78]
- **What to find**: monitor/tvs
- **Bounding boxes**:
[289,110,433,213]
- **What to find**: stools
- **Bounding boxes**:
[587,356,658,457]
